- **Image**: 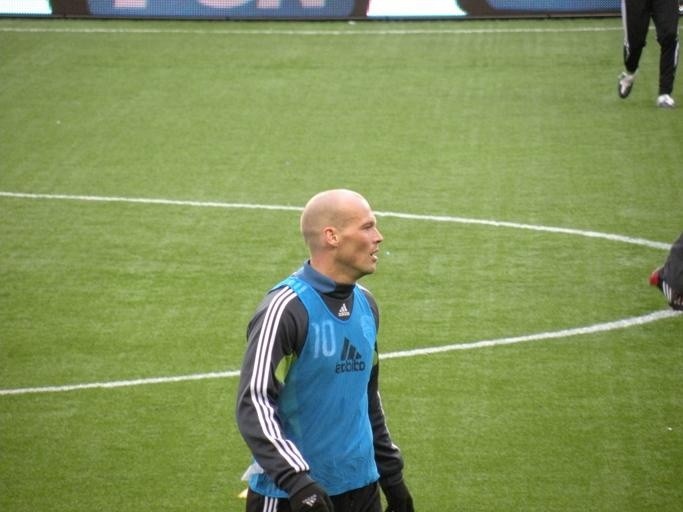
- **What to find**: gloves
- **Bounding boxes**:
[288,482,335,512]
[381,480,414,512]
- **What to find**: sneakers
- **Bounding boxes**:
[656,94,674,108]
[648,264,683,313]
[617,68,638,100]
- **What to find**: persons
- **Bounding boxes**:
[234,188,416,510]
[617,1,680,107]
[656,232,682,310]
